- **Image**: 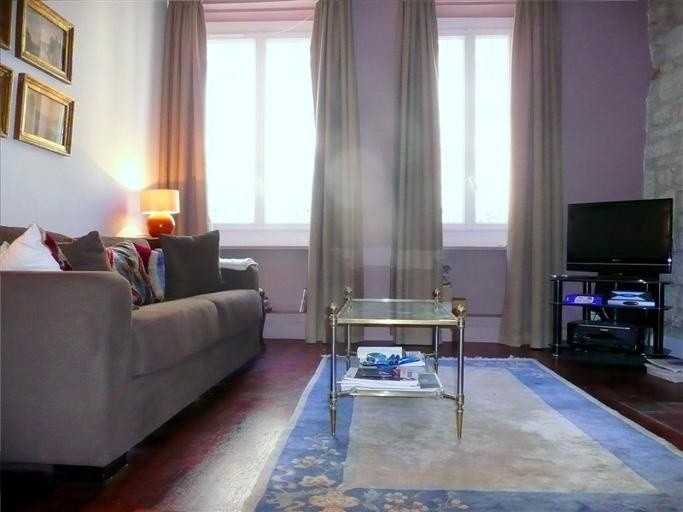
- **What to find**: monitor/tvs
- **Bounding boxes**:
[566,197,673,279]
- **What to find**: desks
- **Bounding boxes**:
[550,271,674,360]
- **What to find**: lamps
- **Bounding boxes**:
[138,182,183,238]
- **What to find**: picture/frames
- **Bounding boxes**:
[0,61,15,139]
[15,72,77,159]
[0,0,13,52]
[18,0,76,86]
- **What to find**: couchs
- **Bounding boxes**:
[0,221,272,494]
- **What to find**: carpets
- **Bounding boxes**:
[242,349,682,511]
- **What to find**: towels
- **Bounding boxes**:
[217,255,259,275]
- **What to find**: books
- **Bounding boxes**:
[645,354,683,384]
[335,343,447,395]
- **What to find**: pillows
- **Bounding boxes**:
[1,222,74,273]
[60,229,115,273]
[157,229,224,295]
[133,241,167,305]
[104,238,153,310]
